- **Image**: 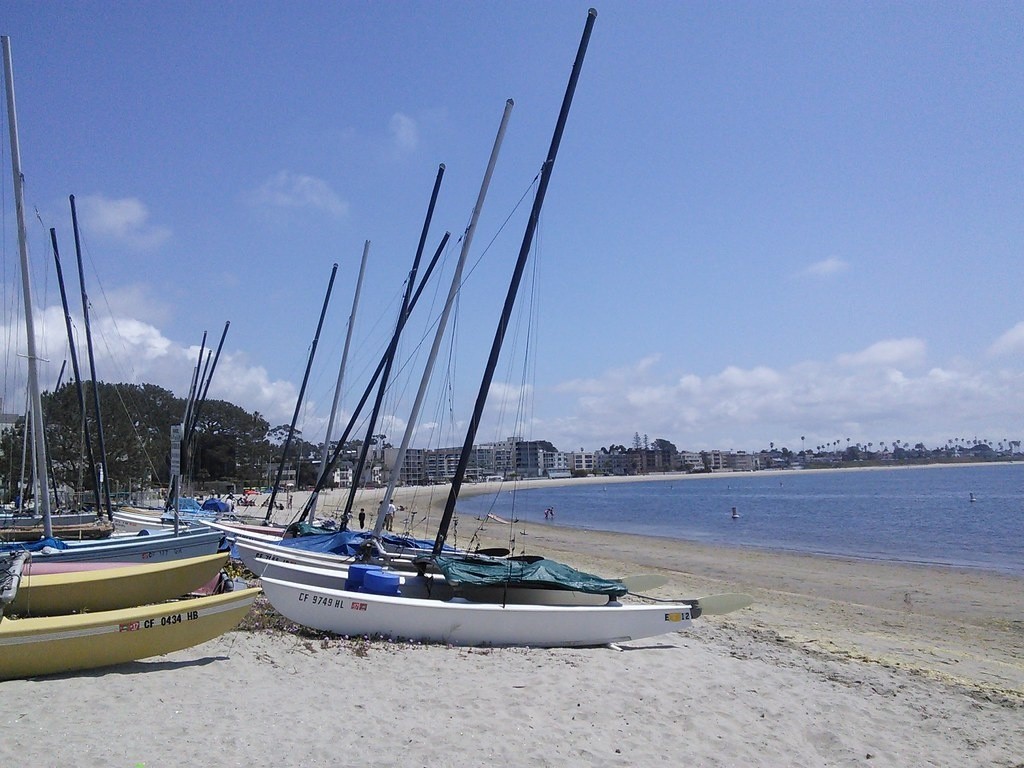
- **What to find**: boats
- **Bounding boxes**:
[0,551,231,619]
[1,586,262,682]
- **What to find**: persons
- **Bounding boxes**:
[359,508,365,529]
[545,504,555,520]
[386,499,396,532]
[378,500,387,528]
[162,490,293,510]
[15,492,27,509]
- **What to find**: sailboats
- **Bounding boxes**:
[257,7,755,649]
[252,99,670,606]
[0,36,512,575]
